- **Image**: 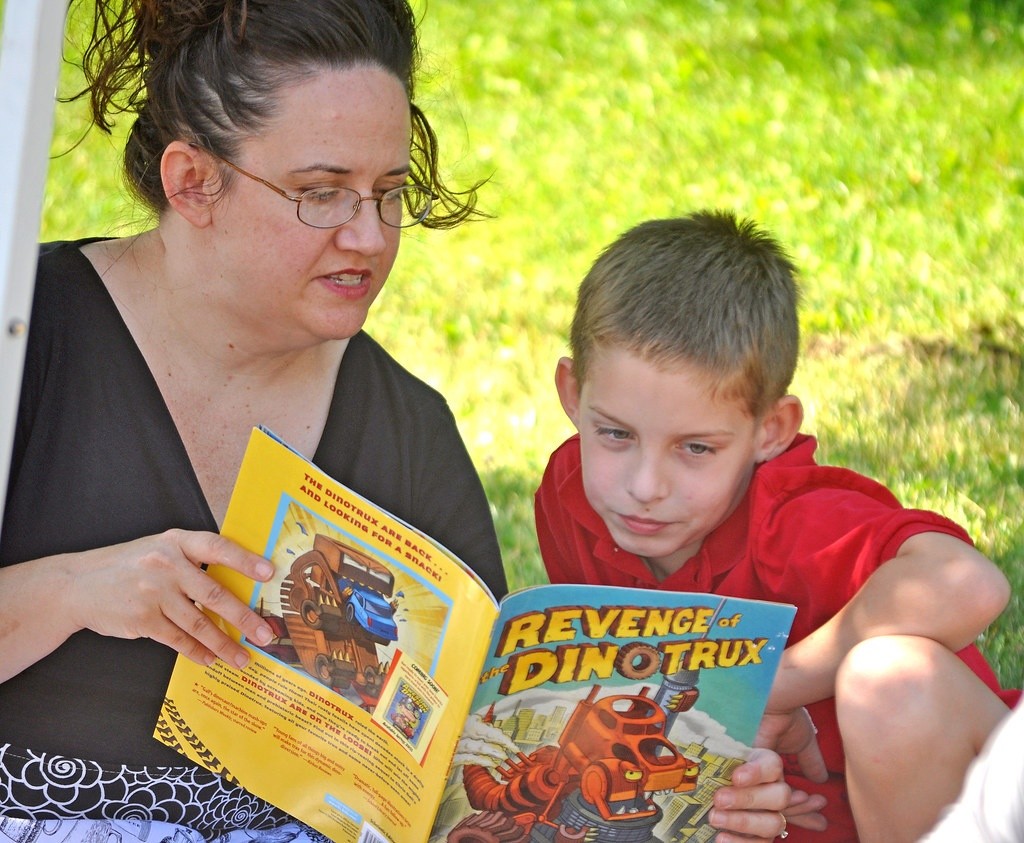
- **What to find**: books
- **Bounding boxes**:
[154,424,798,838]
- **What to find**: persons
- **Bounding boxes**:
[532,210,1024,843]
[0,0,791,843]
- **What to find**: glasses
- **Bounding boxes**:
[185,141,440,230]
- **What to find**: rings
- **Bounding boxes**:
[780,813,789,841]
